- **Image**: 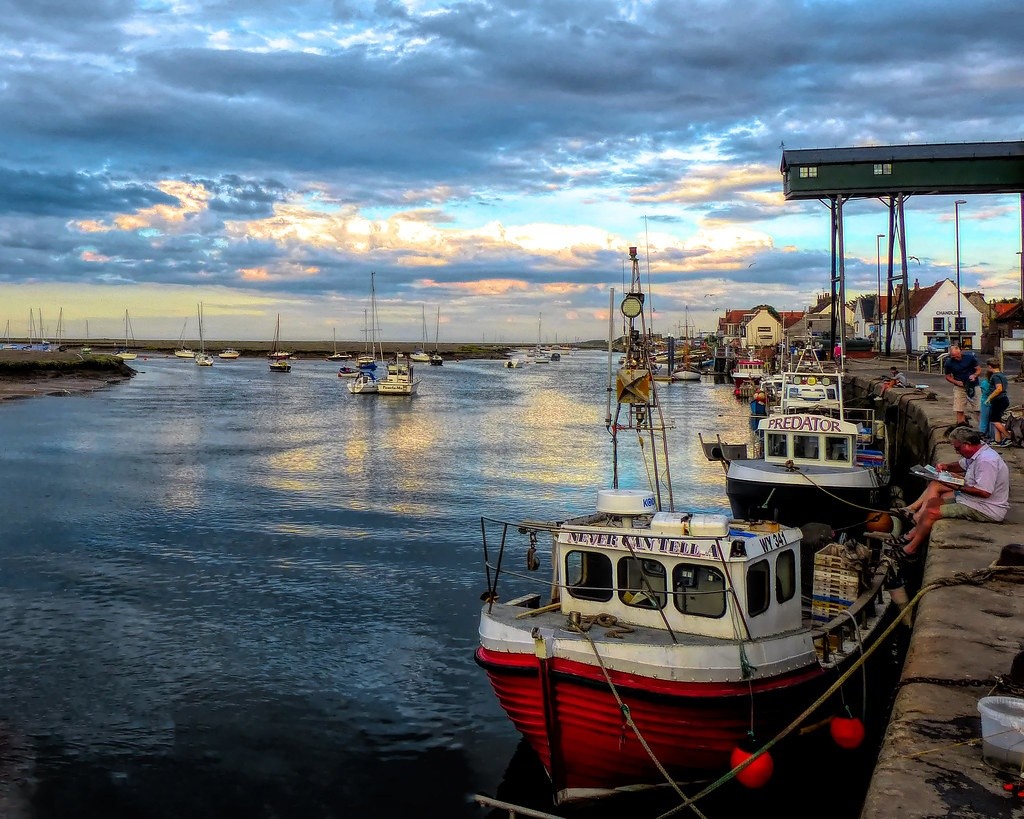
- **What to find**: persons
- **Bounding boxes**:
[834,344,841,365]
[945,346,981,426]
[890,422,988,526]
[978,370,992,436]
[874,367,906,401]
[985,357,1012,447]
[884,426,1009,559]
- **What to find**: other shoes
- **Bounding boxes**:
[874,396,883,400]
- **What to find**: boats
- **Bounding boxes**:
[219,348,240,359]
[375,352,422,396]
[475,245,908,808]
[527,312,572,363]
[618,302,901,534]
[327,351,353,361]
[502,358,524,368]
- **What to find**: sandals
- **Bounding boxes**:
[884,548,918,566]
[883,533,909,546]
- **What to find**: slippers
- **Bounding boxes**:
[890,508,904,521]
[898,508,916,526]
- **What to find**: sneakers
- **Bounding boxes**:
[988,440,1001,446]
[1000,438,1012,447]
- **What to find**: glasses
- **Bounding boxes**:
[954,443,963,451]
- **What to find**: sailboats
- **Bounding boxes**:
[409,302,430,363]
[0,305,93,355]
[268,313,291,372]
[111,308,138,360]
[338,270,384,393]
[194,300,215,366]
[265,324,293,359]
[429,305,444,366]
[174,315,197,357]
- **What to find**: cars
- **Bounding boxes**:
[928,337,949,353]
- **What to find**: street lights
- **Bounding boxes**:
[956,200,968,352]
[877,234,885,354]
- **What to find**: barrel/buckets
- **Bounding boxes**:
[861,428,872,443]
[873,420,884,439]
[977,696,1024,775]
[791,346,797,355]
